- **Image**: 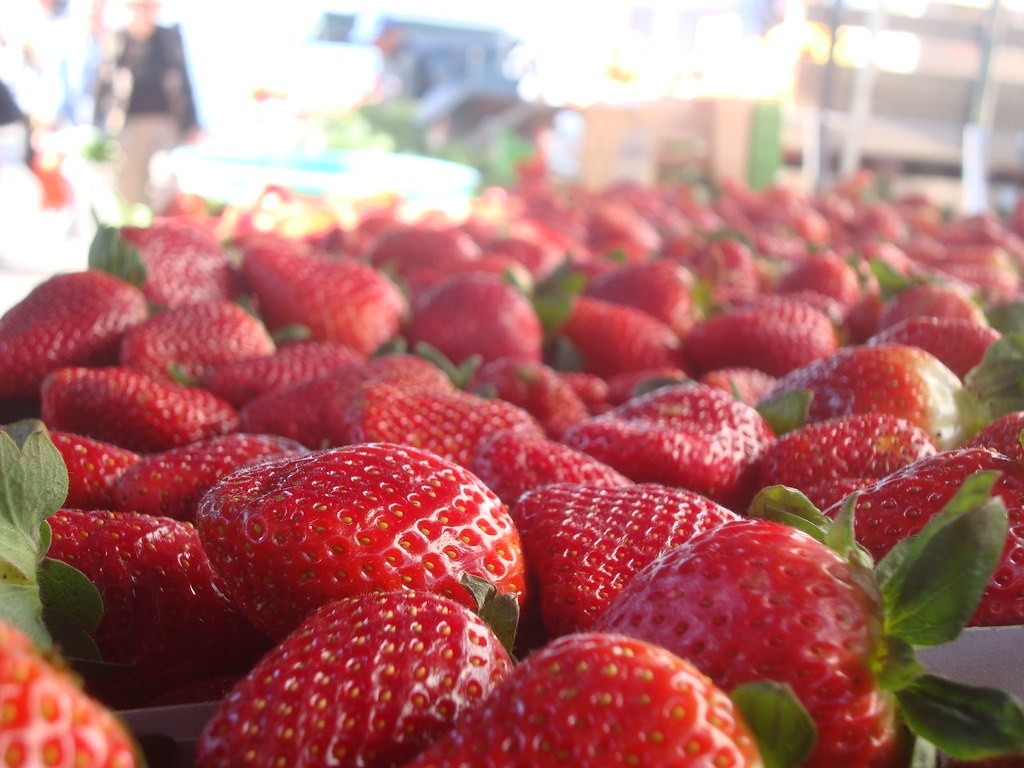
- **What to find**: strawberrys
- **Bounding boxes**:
[0,178,1024,768]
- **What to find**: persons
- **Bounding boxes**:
[93,0,203,215]
